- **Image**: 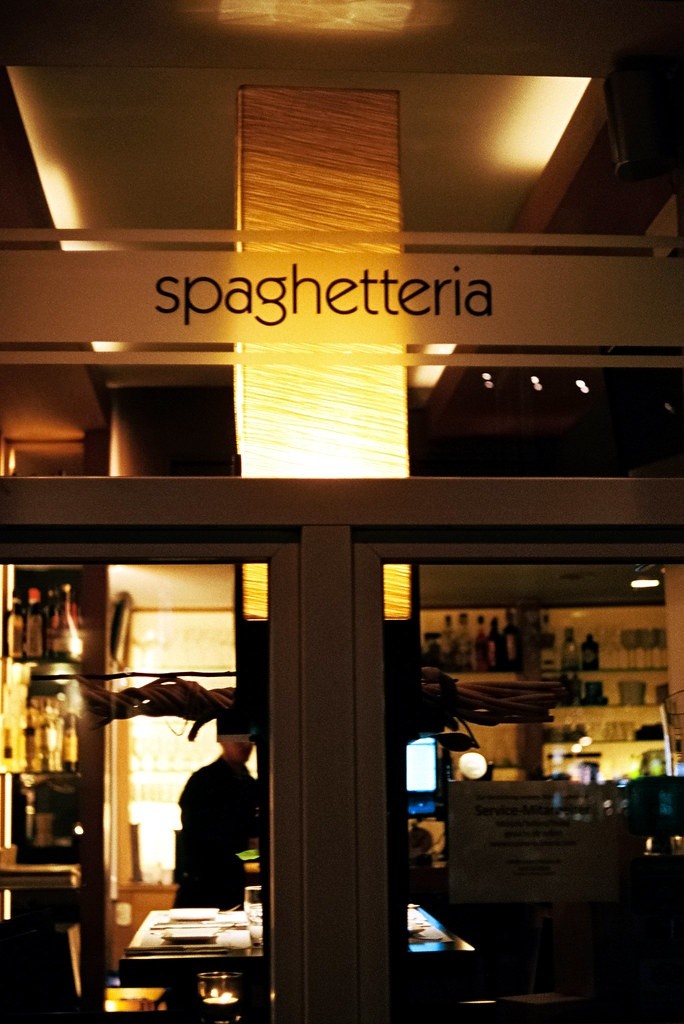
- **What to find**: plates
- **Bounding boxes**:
[160,924,219,944]
[169,908,220,920]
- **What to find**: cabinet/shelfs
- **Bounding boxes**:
[419,602,669,784]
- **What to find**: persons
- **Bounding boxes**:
[175,741,260,907]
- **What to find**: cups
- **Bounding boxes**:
[197,971,244,1024]
[619,680,646,705]
[244,885,264,950]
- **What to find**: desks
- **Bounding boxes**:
[118,912,479,989]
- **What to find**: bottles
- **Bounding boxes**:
[561,626,580,671]
[539,613,559,672]
[6,582,87,663]
[580,633,599,671]
[424,609,524,672]
[0,686,81,774]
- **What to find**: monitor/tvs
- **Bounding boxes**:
[404,737,439,793]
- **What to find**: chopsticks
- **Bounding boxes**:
[150,922,248,930]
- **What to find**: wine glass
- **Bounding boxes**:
[620,628,668,671]
